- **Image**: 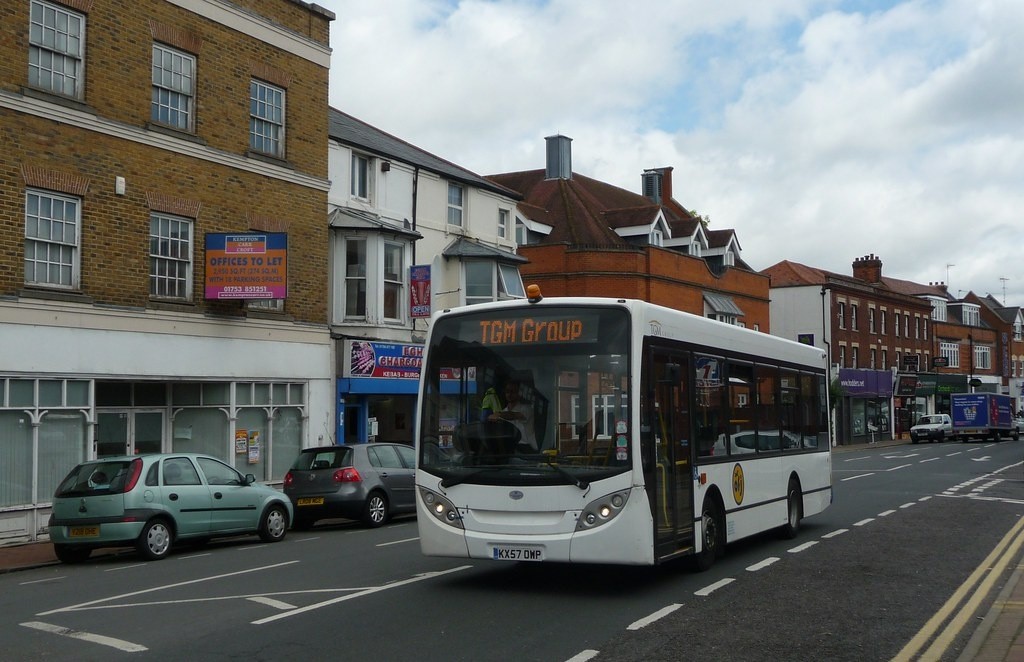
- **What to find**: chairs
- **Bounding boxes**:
[313,460,330,469]
[164,463,185,484]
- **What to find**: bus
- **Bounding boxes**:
[412,283,833,573]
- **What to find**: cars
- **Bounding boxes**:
[709,427,811,456]
[1013,413,1024,435]
[909,413,953,444]
[282,441,417,528]
[47,451,295,561]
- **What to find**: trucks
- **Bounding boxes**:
[952,391,1020,442]
